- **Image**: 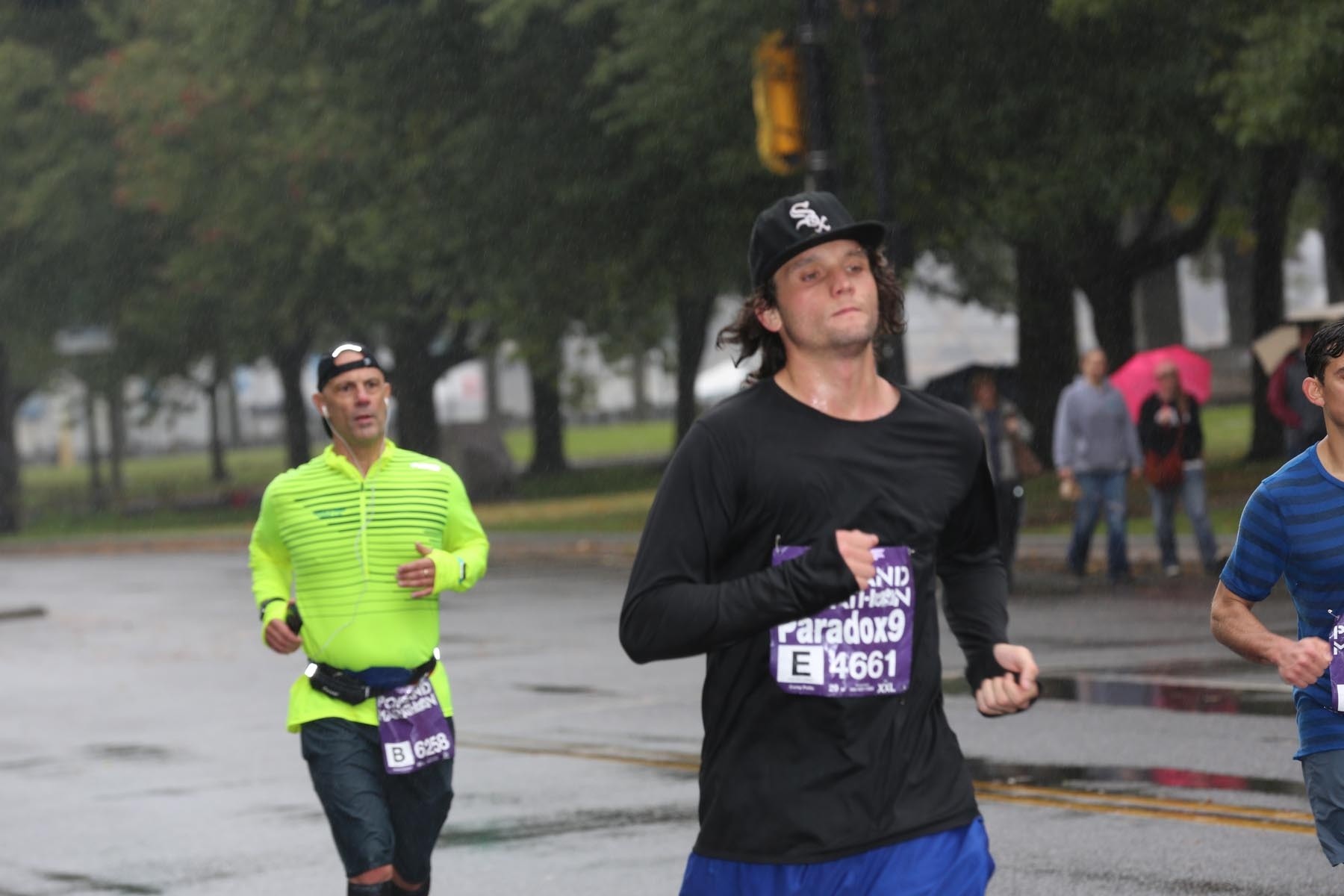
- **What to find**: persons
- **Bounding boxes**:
[1209,321,1344,867]
[1265,321,1328,461]
[619,190,1042,896]
[965,371,1034,591]
[1136,360,1220,577]
[249,340,489,896]
[1053,349,1145,583]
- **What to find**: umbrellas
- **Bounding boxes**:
[925,361,1045,412]
[1252,301,1344,376]
[1110,344,1212,424]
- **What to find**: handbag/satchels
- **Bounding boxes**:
[1010,431,1044,479]
[1143,446,1184,491]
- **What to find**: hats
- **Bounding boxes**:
[745,193,892,285]
[318,340,388,438]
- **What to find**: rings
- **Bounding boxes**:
[424,568,429,577]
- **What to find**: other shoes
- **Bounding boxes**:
[1166,564,1181,578]
[1065,554,1087,577]
[1206,556,1230,576]
[1110,570,1141,580]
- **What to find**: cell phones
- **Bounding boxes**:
[287,604,303,635]
